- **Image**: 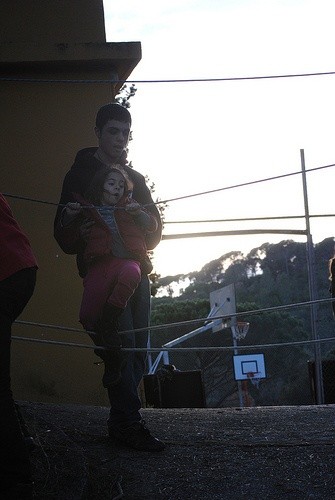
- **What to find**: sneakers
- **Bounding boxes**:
[108,418,167,451]
[95,302,126,349]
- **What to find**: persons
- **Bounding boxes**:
[57,165,159,387]
[0,192,41,500]
[54,101,169,453]
